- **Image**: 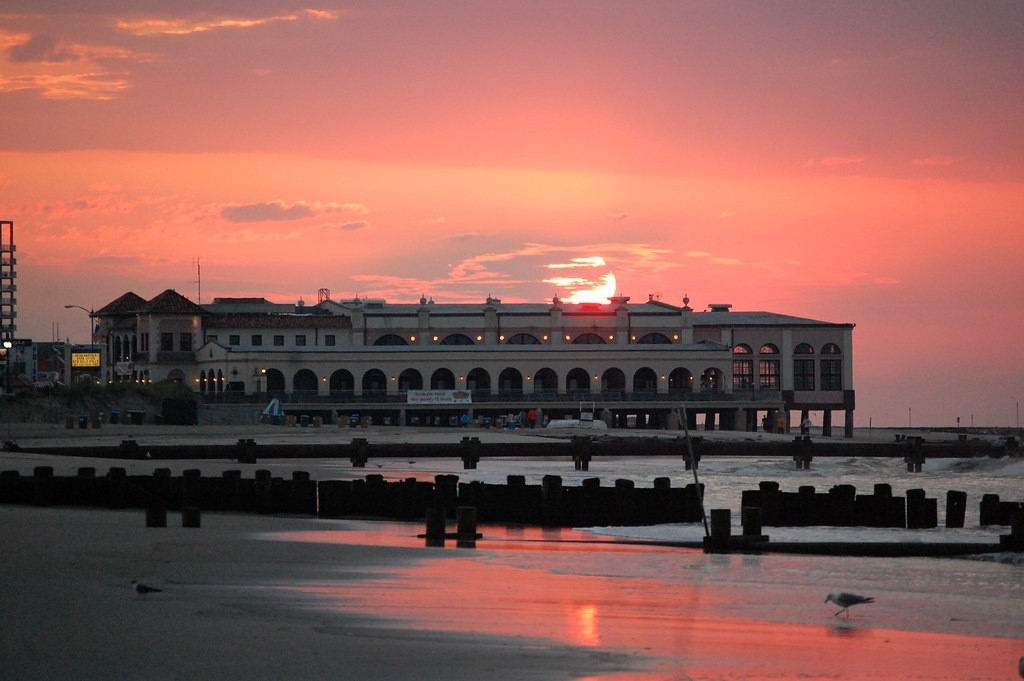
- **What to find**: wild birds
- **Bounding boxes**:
[129,580,162,599]
[824,592,875,618]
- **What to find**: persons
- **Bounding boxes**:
[800,417,812,435]
[520,410,526,427]
[529,410,538,429]
[761,415,767,429]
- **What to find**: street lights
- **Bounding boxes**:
[4,332,12,395]
[64,304,94,354]
